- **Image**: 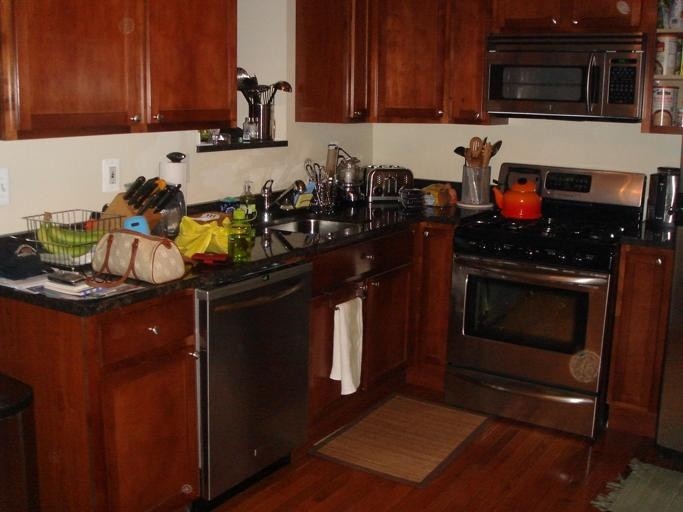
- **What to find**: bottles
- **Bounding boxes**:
[199,130,230,147]
[151,186,186,238]
[227,207,251,262]
[242,116,258,141]
[219,188,274,224]
[325,144,336,176]
[262,227,272,249]
[651,0,683,128]
[343,185,360,202]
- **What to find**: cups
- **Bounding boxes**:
[247,102,272,141]
[461,165,490,204]
[311,182,337,217]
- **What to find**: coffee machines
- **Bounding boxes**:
[646,165,683,233]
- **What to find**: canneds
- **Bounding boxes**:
[652,86,679,127]
[653,34,681,77]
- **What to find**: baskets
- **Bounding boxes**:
[23,210,126,273]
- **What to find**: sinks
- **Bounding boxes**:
[267,213,357,234]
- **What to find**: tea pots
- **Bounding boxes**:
[492,178,543,219]
[335,146,363,184]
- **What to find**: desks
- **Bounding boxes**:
[0,368,44,510]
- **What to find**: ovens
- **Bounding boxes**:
[440,254,612,438]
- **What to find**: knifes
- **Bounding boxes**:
[124,176,182,215]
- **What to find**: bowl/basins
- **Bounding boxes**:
[398,189,425,212]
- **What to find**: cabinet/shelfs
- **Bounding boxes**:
[371,0,446,123]
[604,242,682,443]
[0,287,203,510]
[307,226,404,445]
[488,2,646,33]
[442,0,510,128]
[404,220,451,399]
[640,0,682,135]
[293,0,368,124]
[0,0,239,142]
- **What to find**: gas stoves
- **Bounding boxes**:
[453,199,639,274]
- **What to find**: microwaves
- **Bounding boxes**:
[482,35,647,123]
[365,164,413,203]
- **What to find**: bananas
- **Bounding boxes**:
[38,212,104,257]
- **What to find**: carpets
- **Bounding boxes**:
[308,393,492,488]
[589,454,681,512]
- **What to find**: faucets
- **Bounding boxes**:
[260,178,308,216]
[261,226,294,263]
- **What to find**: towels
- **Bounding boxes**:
[326,294,370,397]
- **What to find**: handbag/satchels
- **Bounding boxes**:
[92,227,187,286]
